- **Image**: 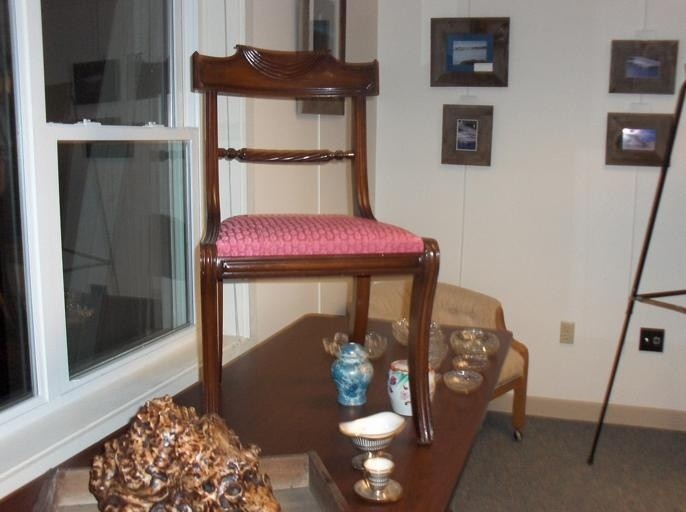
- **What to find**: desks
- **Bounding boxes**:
[0,288,162,398]
[0,312,515,511]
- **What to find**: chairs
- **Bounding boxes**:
[359,281,529,440]
[191,47,443,448]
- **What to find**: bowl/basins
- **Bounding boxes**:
[443,369,485,393]
[450,329,500,357]
[390,317,438,345]
[452,353,489,371]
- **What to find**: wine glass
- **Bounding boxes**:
[337,410,407,471]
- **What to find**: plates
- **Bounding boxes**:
[353,478,402,502]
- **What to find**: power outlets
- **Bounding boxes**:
[560,321,575,346]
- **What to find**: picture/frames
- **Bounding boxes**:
[441,101,493,168]
[605,37,681,97]
[135,58,171,101]
[604,109,676,170]
[292,2,349,115]
[428,16,510,87]
[82,118,136,161]
[69,59,122,103]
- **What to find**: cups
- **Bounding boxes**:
[362,458,394,491]
